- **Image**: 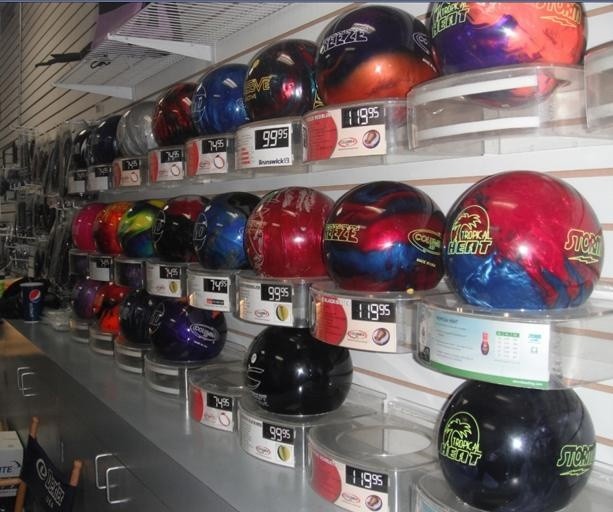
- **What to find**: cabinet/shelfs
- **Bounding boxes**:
[2,332,62,474]
[59,402,173,512]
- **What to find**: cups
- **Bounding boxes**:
[20,282,45,323]
